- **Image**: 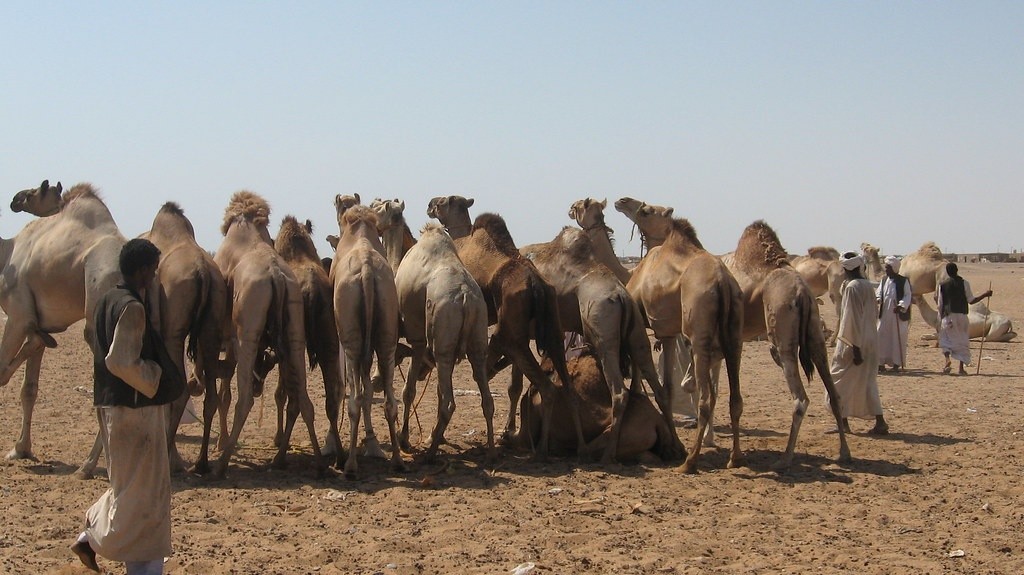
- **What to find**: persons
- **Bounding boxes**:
[70,238,185,575]
[653,334,699,428]
[937,263,993,375]
[825,252,889,434]
[875,256,912,373]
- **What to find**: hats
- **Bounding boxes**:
[839,251,862,272]
[885,256,901,273]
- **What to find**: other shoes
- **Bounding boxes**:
[889,368,899,373]
[878,368,886,372]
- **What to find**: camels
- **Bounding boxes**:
[0,179,1017,481]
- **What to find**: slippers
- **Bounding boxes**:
[823,425,853,434]
[942,367,951,375]
[959,371,966,376]
[867,427,888,434]
